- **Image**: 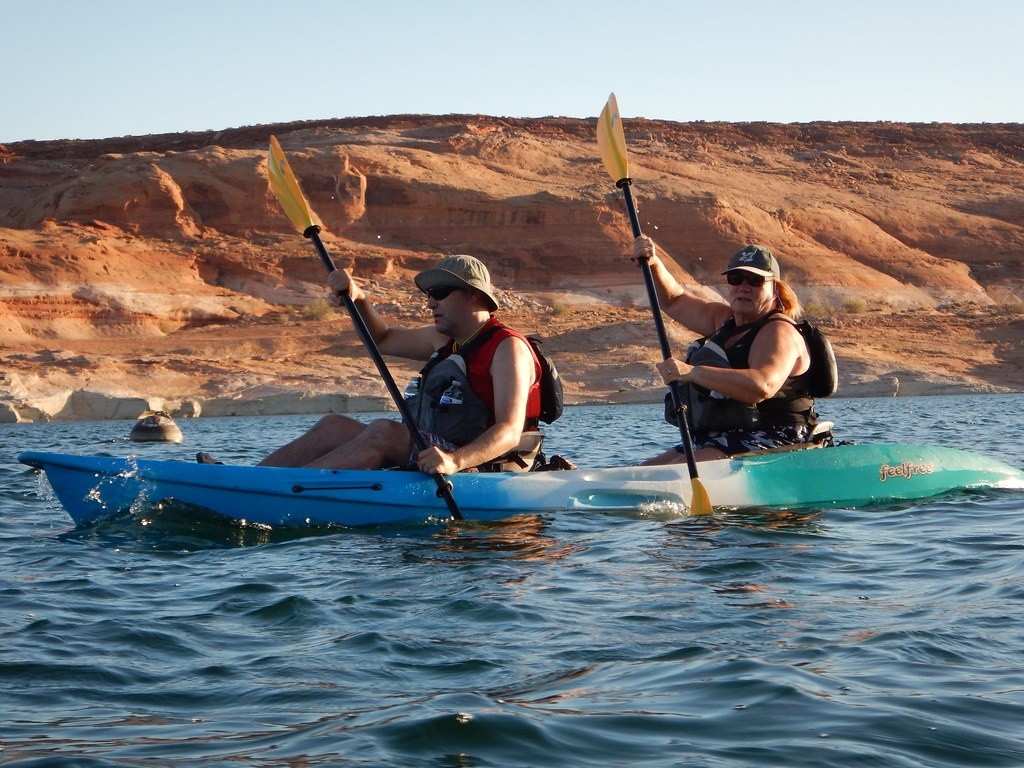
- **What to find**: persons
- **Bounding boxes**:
[630,233,831,467]
[195,256,541,474]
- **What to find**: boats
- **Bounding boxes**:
[15,442,1023,527]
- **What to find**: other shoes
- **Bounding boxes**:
[551,454,578,470]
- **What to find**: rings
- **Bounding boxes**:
[666,368,672,375]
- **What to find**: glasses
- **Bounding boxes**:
[726,272,773,287]
[426,286,462,301]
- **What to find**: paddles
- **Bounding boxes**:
[266,134,463,523]
[596,93,716,518]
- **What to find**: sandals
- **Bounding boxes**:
[196,451,223,464]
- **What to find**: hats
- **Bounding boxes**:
[414,254,499,313]
[721,245,781,280]
